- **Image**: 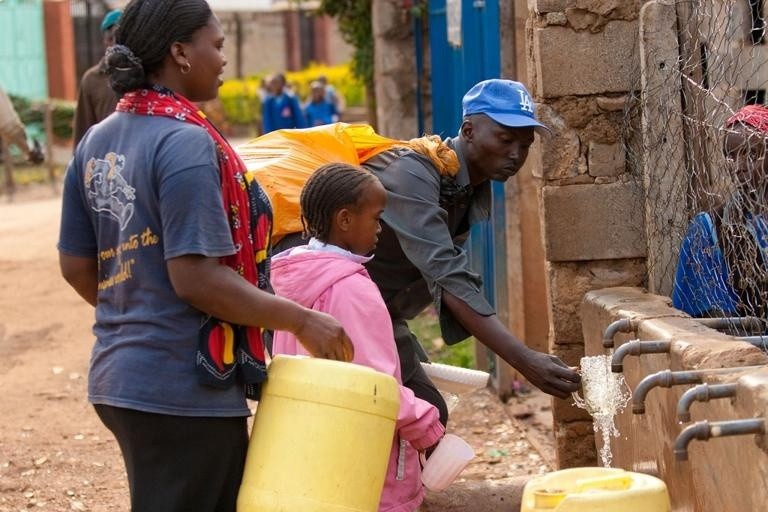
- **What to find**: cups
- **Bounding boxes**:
[567,355,617,416]
[419,433,475,493]
[520,385,527,392]
[513,381,520,389]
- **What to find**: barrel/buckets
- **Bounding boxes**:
[236,355,403,511]
[521,466,670,512]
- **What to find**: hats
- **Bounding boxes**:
[101,9,122,31]
[462,77,553,138]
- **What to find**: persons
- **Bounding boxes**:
[258,71,343,134]
[275,165,445,511]
[57,0,354,511]
[670,106,768,355]
[72,10,123,149]
[280,80,585,476]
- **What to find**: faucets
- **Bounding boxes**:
[629,369,695,414]
[670,425,768,460]
[600,317,635,347]
[609,334,669,372]
[675,382,739,421]
